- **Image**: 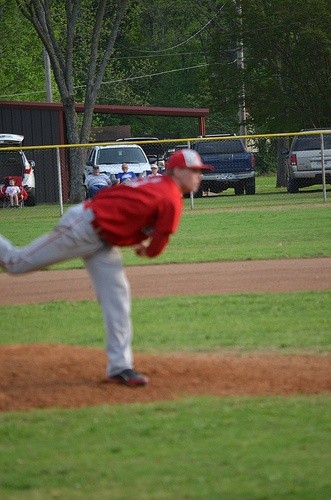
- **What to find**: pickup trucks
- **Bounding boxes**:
[194,134,257,196]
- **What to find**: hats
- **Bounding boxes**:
[167,148,213,174]
[122,163,128,167]
[93,164,99,168]
[151,164,158,169]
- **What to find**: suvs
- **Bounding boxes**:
[83,144,153,203]
[281,128,331,194]
[0,134,37,207]
[115,137,167,161]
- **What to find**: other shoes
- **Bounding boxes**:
[11,204,20,208]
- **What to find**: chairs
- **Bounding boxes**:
[145,170,165,177]
[114,173,142,185]
[82,171,107,200]
[0,176,31,209]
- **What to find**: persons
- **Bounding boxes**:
[0,149,202,388]
[85,162,163,197]
[5,177,21,207]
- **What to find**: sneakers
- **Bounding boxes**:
[108,369,146,386]
